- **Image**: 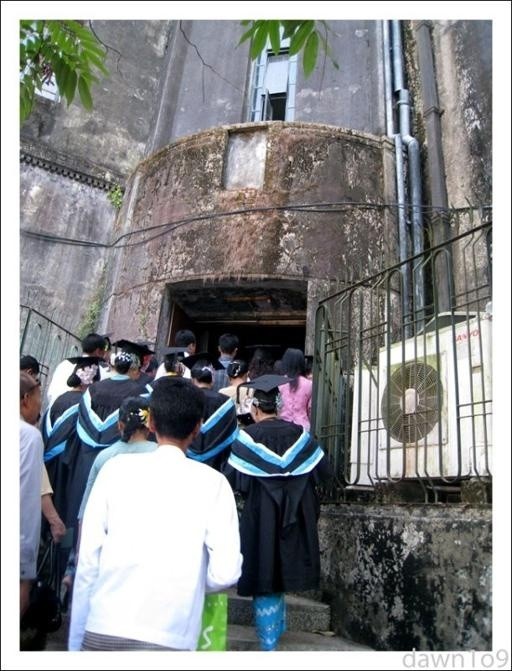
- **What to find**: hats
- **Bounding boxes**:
[304,355,314,369]
[157,347,191,360]
[64,356,106,367]
[245,344,280,359]
[112,339,156,356]
[239,374,296,401]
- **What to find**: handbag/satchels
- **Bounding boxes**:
[196,593,228,651]
[29,536,62,634]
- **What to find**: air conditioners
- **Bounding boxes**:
[375,313,493,480]
